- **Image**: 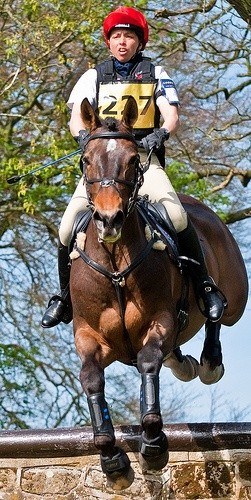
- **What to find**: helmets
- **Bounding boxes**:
[102,7,149,43]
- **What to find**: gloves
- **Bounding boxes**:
[141,128,171,152]
[73,130,90,146]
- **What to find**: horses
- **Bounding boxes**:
[71,94,251,474]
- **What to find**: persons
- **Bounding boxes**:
[39,8,226,331]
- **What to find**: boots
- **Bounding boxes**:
[42,237,70,327]
[177,215,224,322]
